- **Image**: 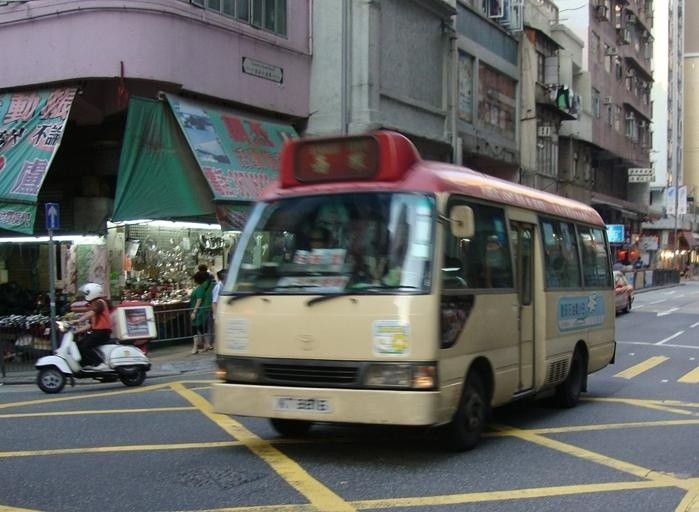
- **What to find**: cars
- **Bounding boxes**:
[613,269,634,317]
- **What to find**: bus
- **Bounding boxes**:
[210,130,617,454]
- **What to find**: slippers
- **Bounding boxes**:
[201,345,212,353]
[189,350,199,355]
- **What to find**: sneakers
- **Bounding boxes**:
[93,363,109,370]
[83,364,94,369]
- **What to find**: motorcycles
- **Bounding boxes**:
[35,301,158,394]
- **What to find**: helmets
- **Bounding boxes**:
[78,282,102,301]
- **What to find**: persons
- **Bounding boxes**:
[68,282,112,371]
[307,227,333,250]
[613,259,627,273]
[683,259,691,279]
[634,256,643,269]
[189,263,229,355]
